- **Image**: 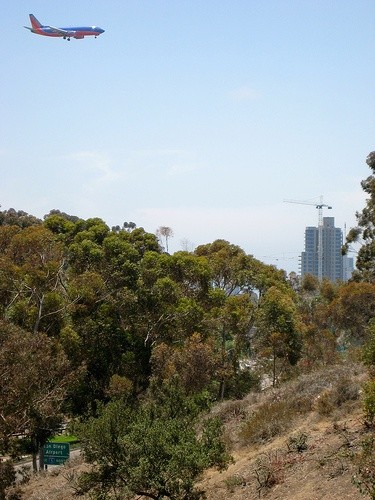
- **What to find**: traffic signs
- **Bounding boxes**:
[41,442,70,466]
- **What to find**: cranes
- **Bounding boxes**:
[283,194,332,281]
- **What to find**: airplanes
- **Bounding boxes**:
[24,12,105,42]
